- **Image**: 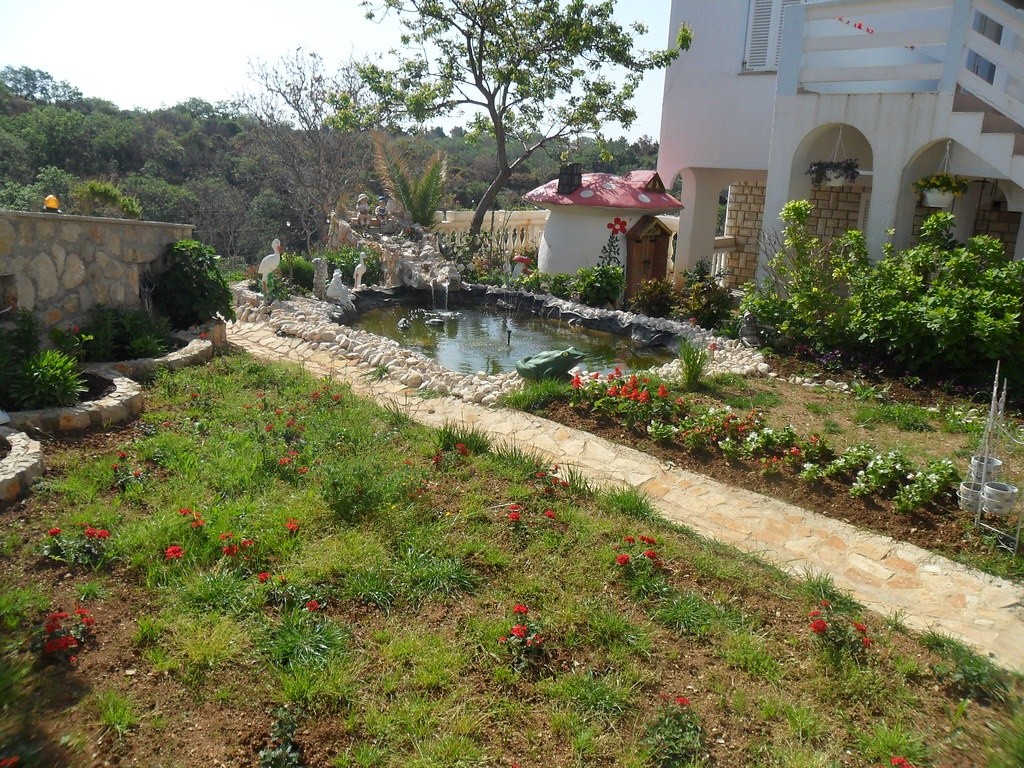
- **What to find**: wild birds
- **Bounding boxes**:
[353,251,367,289]
[258,239,281,300]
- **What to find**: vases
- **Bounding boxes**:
[983,482,1020,514]
[821,169,846,186]
[960,481,984,512]
[919,188,955,208]
[970,456,1002,483]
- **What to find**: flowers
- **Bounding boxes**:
[37,318,955,768]
[910,173,969,200]
[607,217,627,236]
[804,158,860,188]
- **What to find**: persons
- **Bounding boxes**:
[356,193,372,227]
[738,311,762,348]
[375,196,389,229]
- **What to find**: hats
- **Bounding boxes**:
[379,196,386,202]
[357,194,369,202]
